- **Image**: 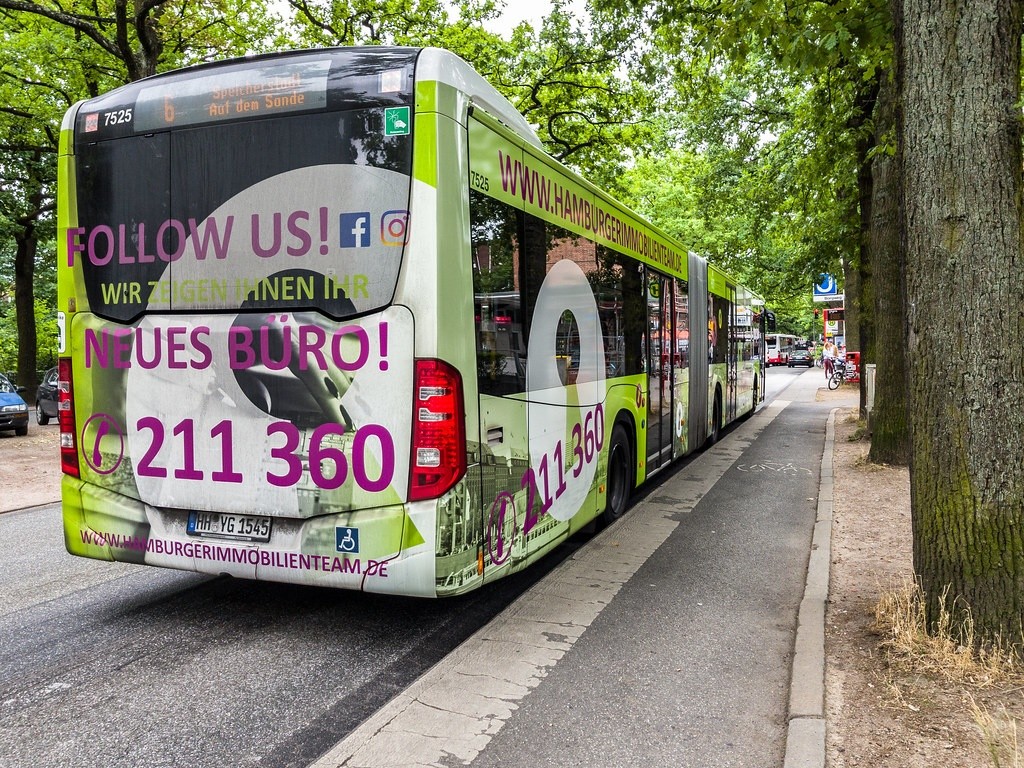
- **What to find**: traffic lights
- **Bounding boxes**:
[815,309,819,319]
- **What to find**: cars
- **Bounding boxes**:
[0,373,29,436]
[787,349,815,368]
[36,366,60,425]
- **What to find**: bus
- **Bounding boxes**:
[56,48,776,598]
[765,334,806,368]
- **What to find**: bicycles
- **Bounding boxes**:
[816,354,846,390]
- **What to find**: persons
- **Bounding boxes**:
[822,340,838,378]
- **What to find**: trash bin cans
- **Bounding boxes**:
[845,352,860,383]
